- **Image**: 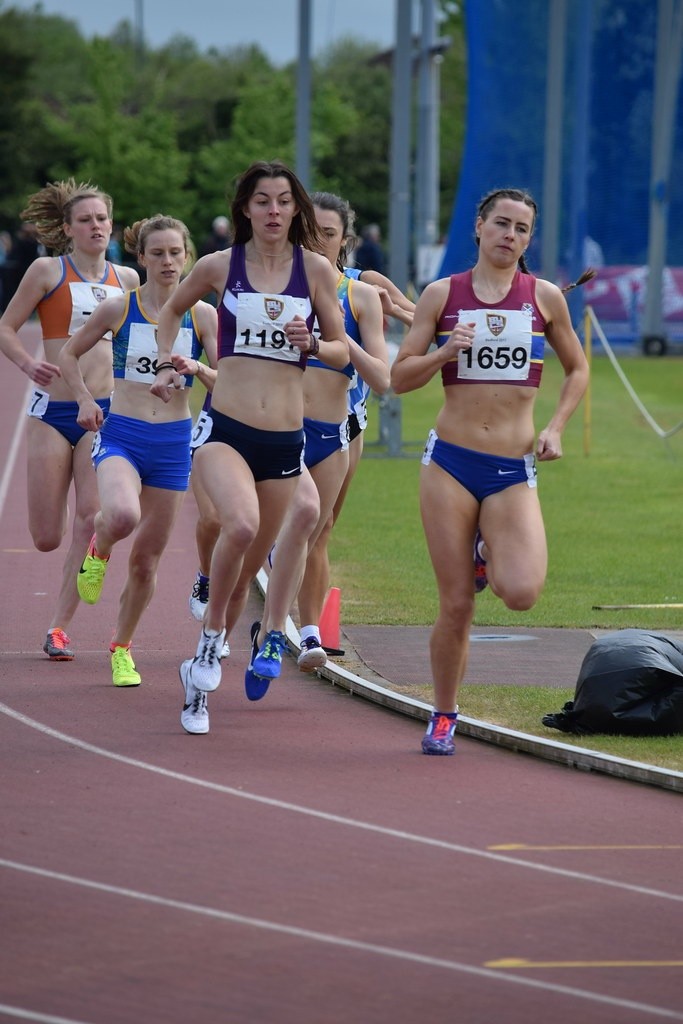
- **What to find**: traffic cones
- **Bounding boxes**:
[320,586,345,656]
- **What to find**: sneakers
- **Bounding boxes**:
[108,631,141,686]
[473,532,489,593]
[421,705,459,755]
[191,625,230,692]
[42,628,75,661]
[253,630,291,680]
[244,621,272,701]
[189,569,209,622]
[77,532,111,605]
[179,658,209,735]
[297,635,328,672]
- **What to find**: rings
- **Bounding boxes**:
[293,327,297,335]
[465,337,469,342]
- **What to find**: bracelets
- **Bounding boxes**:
[306,334,319,356]
[301,333,314,354]
[194,360,200,375]
[155,362,177,375]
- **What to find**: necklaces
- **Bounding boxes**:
[255,250,287,257]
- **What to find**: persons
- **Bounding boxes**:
[190,189,415,698]
[356,223,387,276]
[150,161,351,734]
[204,215,230,308]
[0,220,120,316]
[59,212,217,687]
[390,189,596,755]
[0,176,140,661]
